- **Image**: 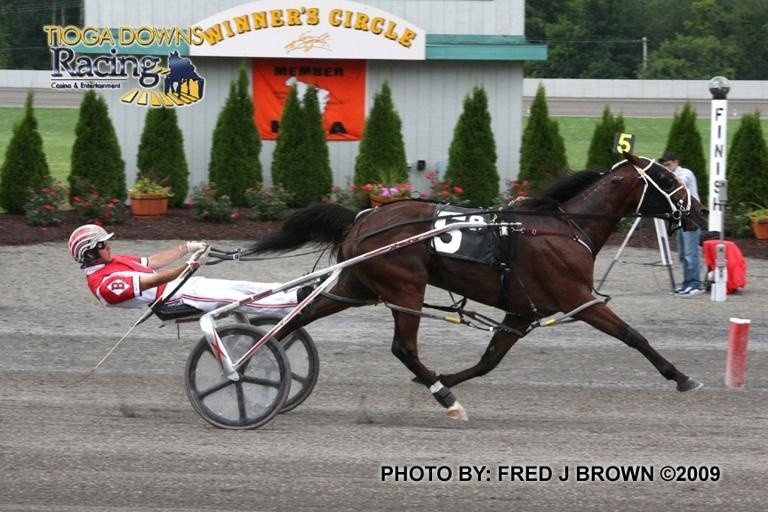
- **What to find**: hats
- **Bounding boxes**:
[659,152,675,164]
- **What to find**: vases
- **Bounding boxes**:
[369,195,406,208]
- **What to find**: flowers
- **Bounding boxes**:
[362,172,469,203]
[504,180,532,205]
[21,176,125,228]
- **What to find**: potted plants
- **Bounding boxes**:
[745,208,768,240]
[128,175,171,217]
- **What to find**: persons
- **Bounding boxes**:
[67,222,330,320]
[657,153,707,298]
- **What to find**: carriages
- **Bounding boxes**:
[149,151,709,429]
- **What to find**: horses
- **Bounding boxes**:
[239,149,712,424]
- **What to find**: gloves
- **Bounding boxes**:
[186,246,211,271]
[188,239,206,251]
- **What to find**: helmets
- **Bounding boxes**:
[68,225,116,264]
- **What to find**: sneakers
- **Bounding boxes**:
[675,285,707,295]
[298,273,331,316]
